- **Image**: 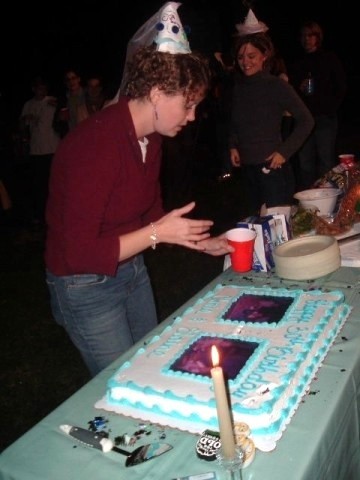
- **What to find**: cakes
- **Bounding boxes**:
[106,282,350,436]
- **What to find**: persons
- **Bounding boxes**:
[0,20,345,230]
[46,48,234,377]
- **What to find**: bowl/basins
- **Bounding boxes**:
[294,189,342,217]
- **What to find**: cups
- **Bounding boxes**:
[339,155,354,163]
[226,228,256,273]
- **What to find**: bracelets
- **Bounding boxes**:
[149,222,157,249]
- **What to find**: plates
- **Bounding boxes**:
[274,235,341,280]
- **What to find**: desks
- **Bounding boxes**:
[0,264,360,480]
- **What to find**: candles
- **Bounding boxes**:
[209,344,236,459]
